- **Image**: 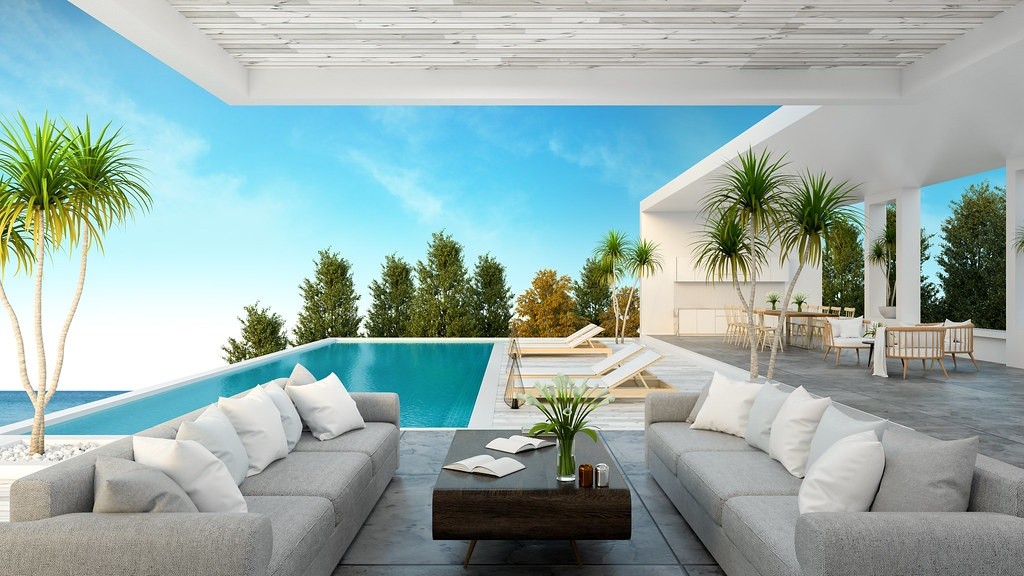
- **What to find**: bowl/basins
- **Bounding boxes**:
[879,306,896,318]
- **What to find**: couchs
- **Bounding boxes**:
[644,369,1024,576]
[0,365,402,576]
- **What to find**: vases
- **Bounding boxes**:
[797,303,802,311]
[554,434,577,481]
[771,300,776,309]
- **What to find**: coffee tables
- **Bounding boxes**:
[432,429,631,568]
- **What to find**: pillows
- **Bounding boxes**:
[747,382,791,453]
[826,318,842,338]
[689,373,763,443]
[283,364,317,393]
[177,404,250,491]
[132,435,251,513]
[805,407,888,480]
[769,385,832,479]
[251,380,303,454]
[944,318,971,342]
[799,430,884,517]
[840,316,863,338]
[219,384,288,476]
[92,456,198,513]
[288,374,364,439]
[875,430,981,516]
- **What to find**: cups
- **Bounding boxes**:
[595,463,609,487]
[579,464,593,486]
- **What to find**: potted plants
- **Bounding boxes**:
[869,223,896,320]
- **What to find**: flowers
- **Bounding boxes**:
[765,293,780,303]
[863,322,883,337]
[512,375,615,441]
[790,292,808,305]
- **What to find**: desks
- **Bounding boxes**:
[863,340,904,370]
[743,309,840,350]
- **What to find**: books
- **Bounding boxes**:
[441,455,526,477]
[484,435,556,453]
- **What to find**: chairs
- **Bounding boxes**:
[822,320,870,367]
[790,304,856,349]
[928,323,980,371]
[870,326,948,379]
[503,323,678,409]
[723,307,783,353]
